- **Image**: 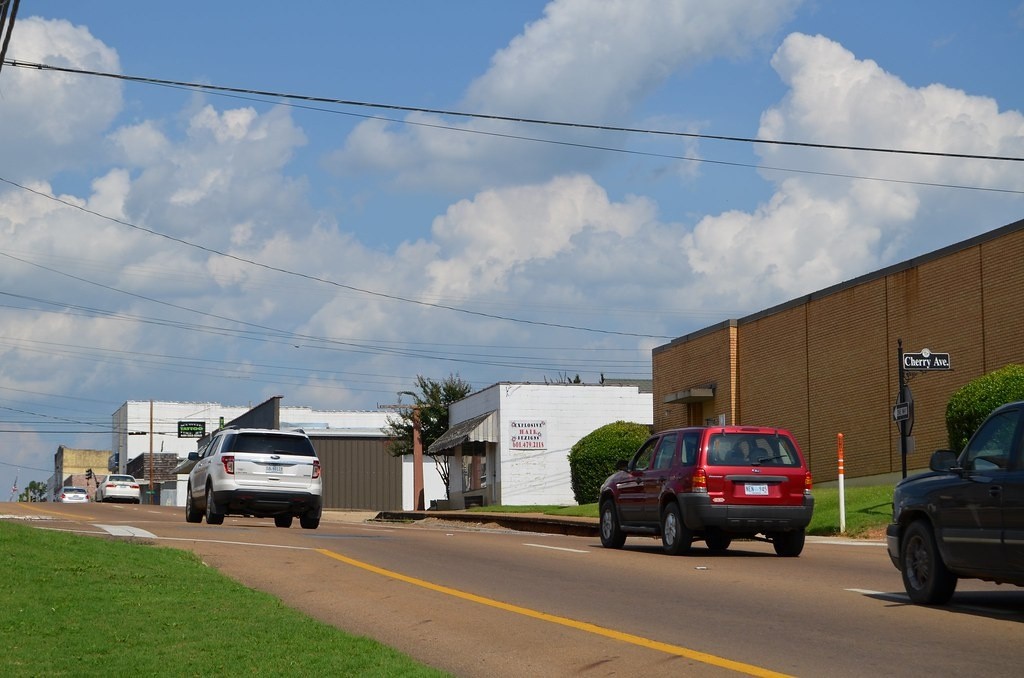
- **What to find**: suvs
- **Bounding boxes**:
[185,425,323,529]
[598,425,814,558]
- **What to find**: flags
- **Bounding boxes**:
[11,479,17,494]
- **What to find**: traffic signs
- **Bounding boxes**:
[892,402,909,422]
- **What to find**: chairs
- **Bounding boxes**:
[725,448,744,463]
[749,449,768,463]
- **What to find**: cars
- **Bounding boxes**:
[884,399,1024,606]
[52,486,89,504]
[94,473,141,505]
